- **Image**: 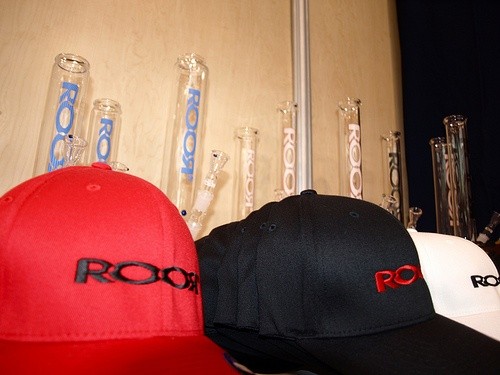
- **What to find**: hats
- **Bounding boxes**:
[0,162,242,375]
[194,189,500,375]
